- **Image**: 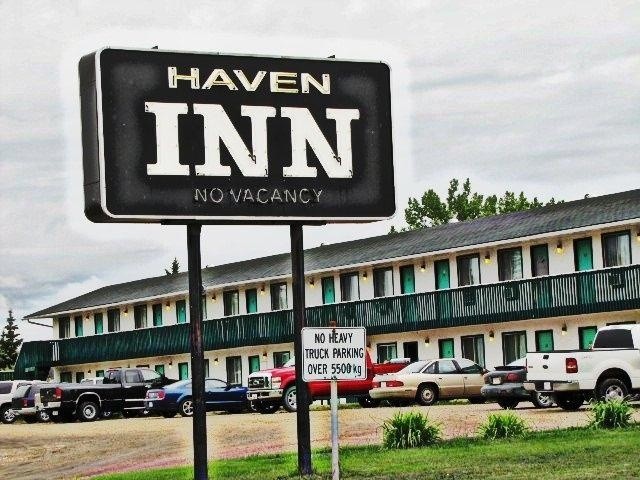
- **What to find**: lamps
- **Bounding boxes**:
[562,324,567,335]
[85,301,172,374]
[359,253,494,352]
[636,232,640,241]
[556,243,562,253]
[211,279,314,363]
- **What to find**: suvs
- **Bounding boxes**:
[1,367,248,424]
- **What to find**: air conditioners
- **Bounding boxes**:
[503,287,517,300]
[608,274,625,286]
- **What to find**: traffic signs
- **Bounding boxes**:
[300,326,366,381]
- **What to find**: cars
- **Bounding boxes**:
[369,356,496,406]
[479,356,556,409]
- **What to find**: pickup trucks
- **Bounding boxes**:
[522,319,640,408]
[246,349,415,414]
